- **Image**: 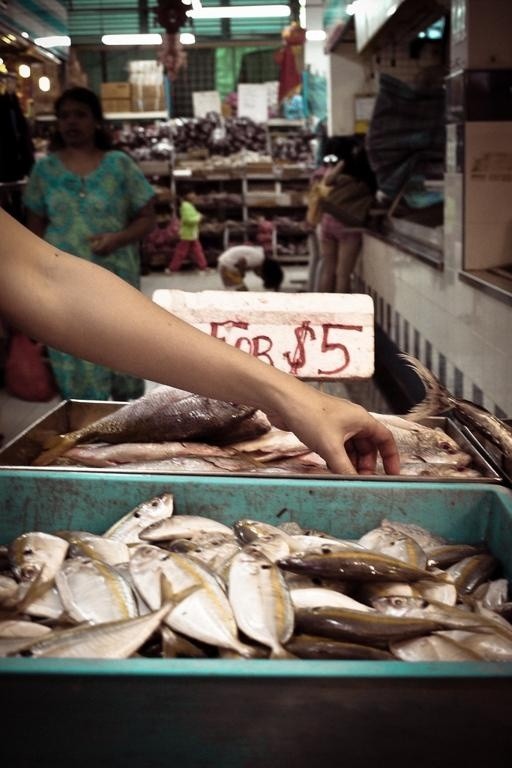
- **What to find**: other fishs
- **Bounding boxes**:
[0,490,512,662]
[32,383,511,478]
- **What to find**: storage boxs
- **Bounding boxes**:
[131,81,165,113]
[99,81,130,99]
[102,99,130,114]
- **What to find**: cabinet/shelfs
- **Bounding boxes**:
[109,120,318,263]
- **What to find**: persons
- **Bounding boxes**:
[1,202,402,479]
[0,89,35,226]
[217,245,284,292]
[302,135,349,292]
[163,186,214,275]
[25,84,157,404]
[322,133,378,292]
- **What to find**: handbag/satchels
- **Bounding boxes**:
[306,181,322,224]
[3,334,55,402]
[321,179,374,225]
